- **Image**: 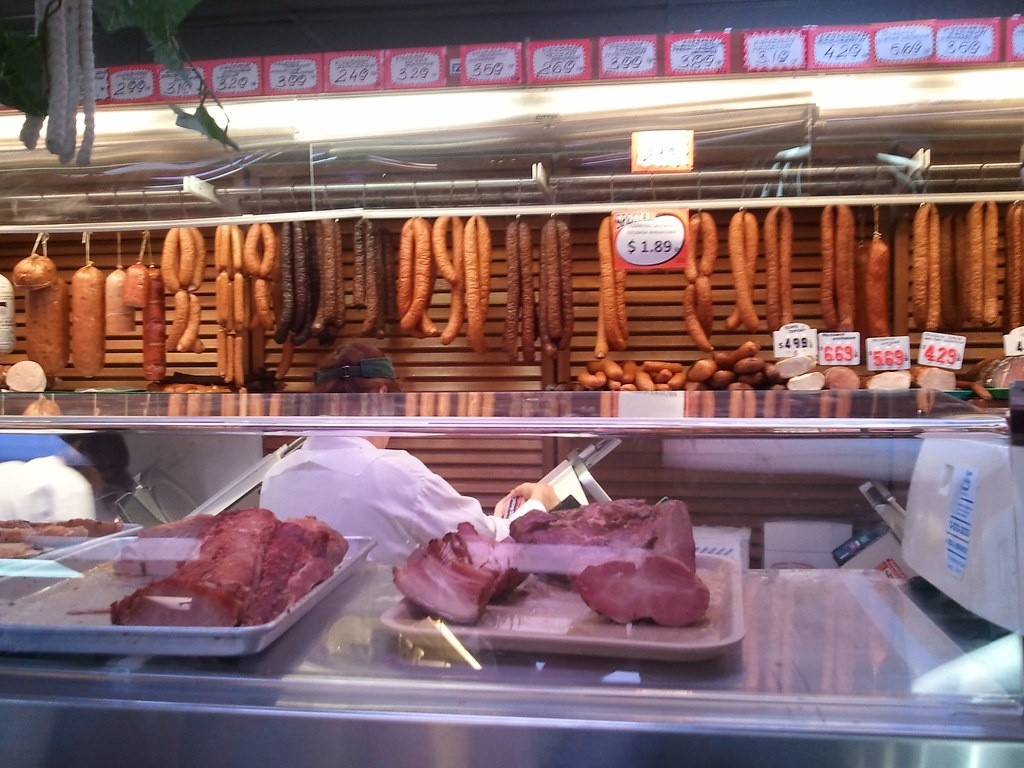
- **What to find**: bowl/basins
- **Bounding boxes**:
[943,389,973,400]
[985,388,1010,399]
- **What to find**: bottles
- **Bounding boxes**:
[0,275,16,354]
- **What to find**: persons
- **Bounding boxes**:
[259,343,562,565]
[0,432,134,523]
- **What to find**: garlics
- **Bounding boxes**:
[20,0,96,168]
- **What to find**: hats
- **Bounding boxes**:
[312,355,405,402]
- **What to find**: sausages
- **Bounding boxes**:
[393,202,1024,399]
[167,391,938,416]
[0,212,387,389]
